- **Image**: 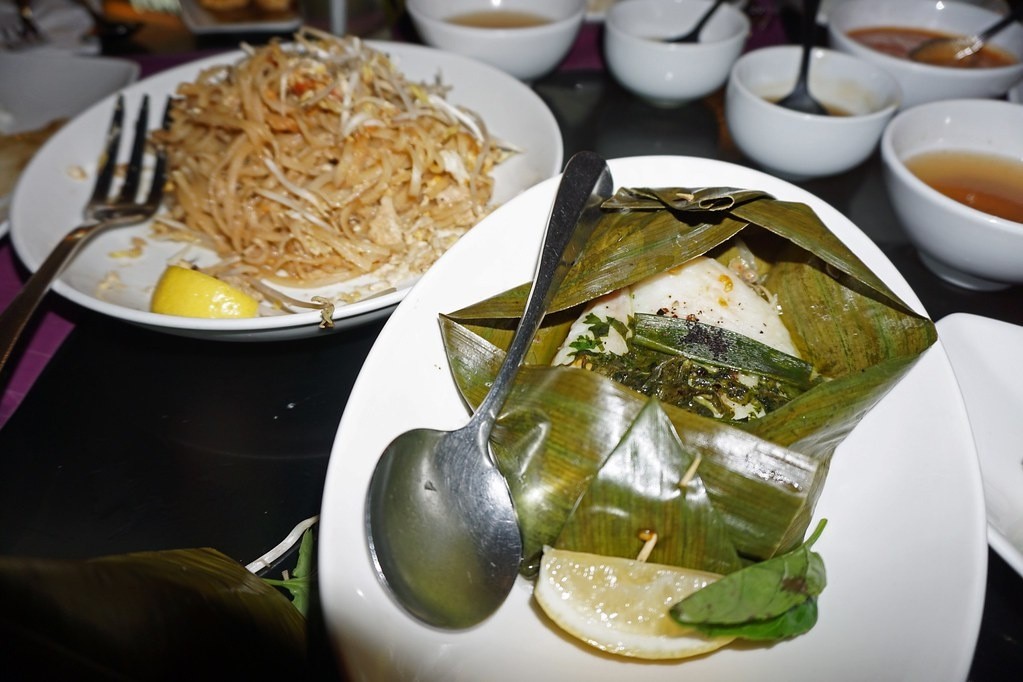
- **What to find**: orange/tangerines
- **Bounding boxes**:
[533,546,759,659]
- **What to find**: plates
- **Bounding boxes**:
[930,308,1023,584]
[316,153,991,681]
[12,35,567,346]
[179,0,305,35]
[0,49,143,245]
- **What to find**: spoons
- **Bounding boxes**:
[366,144,611,628]
[775,27,828,115]
[669,0,721,43]
[908,8,1023,67]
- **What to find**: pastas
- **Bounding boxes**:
[152,27,499,284]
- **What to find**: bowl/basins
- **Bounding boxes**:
[601,1,752,109]
[826,0,1023,113]
[880,94,1023,294]
[724,45,900,183]
[403,0,587,90]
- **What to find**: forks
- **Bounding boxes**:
[0,92,179,366]
